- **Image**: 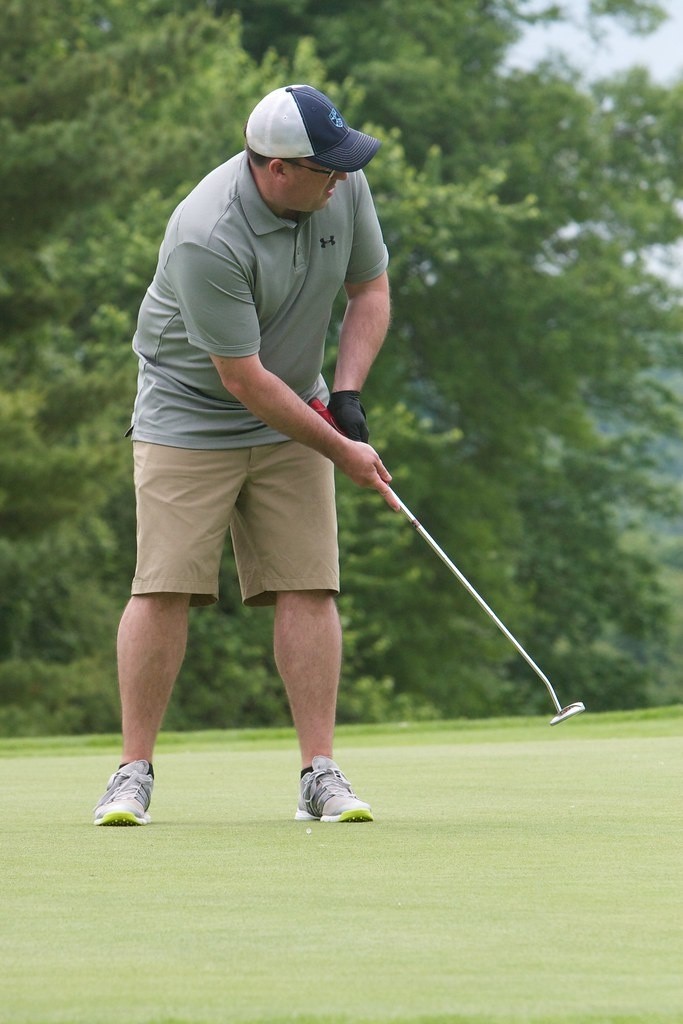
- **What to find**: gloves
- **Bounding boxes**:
[326,390,369,444]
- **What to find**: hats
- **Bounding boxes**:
[245,85,383,172]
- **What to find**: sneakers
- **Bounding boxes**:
[92,759,155,825]
[295,755,374,821]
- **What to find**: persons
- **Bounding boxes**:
[95,85,391,827]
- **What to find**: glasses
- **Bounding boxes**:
[282,158,336,179]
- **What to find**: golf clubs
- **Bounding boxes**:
[307,396,586,726]
